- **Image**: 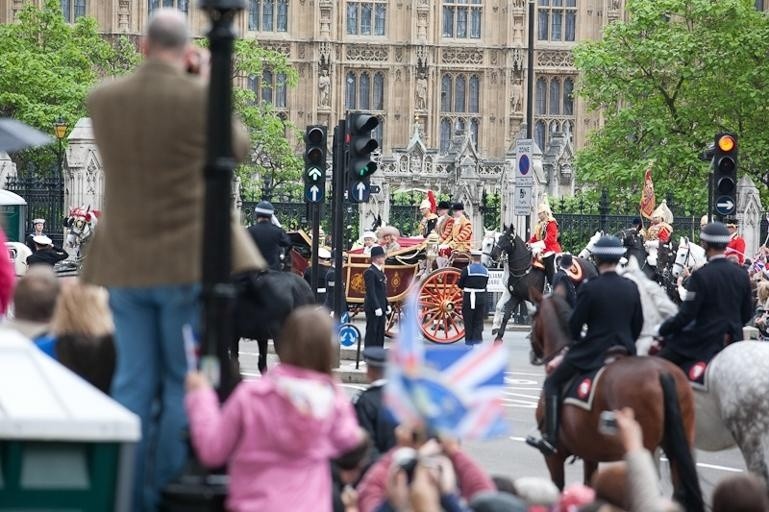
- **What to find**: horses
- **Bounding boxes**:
[610,253,769,480]
[325,231,334,247]
[481,224,707,345]
[66,204,99,275]
[230,268,316,376]
[526,282,713,512]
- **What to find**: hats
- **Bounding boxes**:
[538,204,546,213]
[560,255,573,267]
[318,248,331,261]
[33,219,52,245]
[371,246,384,257]
[255,201,274,216]
[654,209,665,220]
[363,227,400,238]
[700,220,737,243]
[419,200,464,209]
[363,347,388,367]
[591,237,627,256]
[471,250,483,256]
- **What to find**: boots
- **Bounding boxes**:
[527,393,559,455]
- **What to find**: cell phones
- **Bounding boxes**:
[598,411,619,435]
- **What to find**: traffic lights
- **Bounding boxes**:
[342,120,349,189]
[305,126,328,163]
[349,112,379,177]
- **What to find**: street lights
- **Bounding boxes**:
[715,132,738,195]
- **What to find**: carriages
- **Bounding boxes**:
[63,202,470,343]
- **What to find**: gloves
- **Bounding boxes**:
[386,305,392,315]
[375,308,382,316]
